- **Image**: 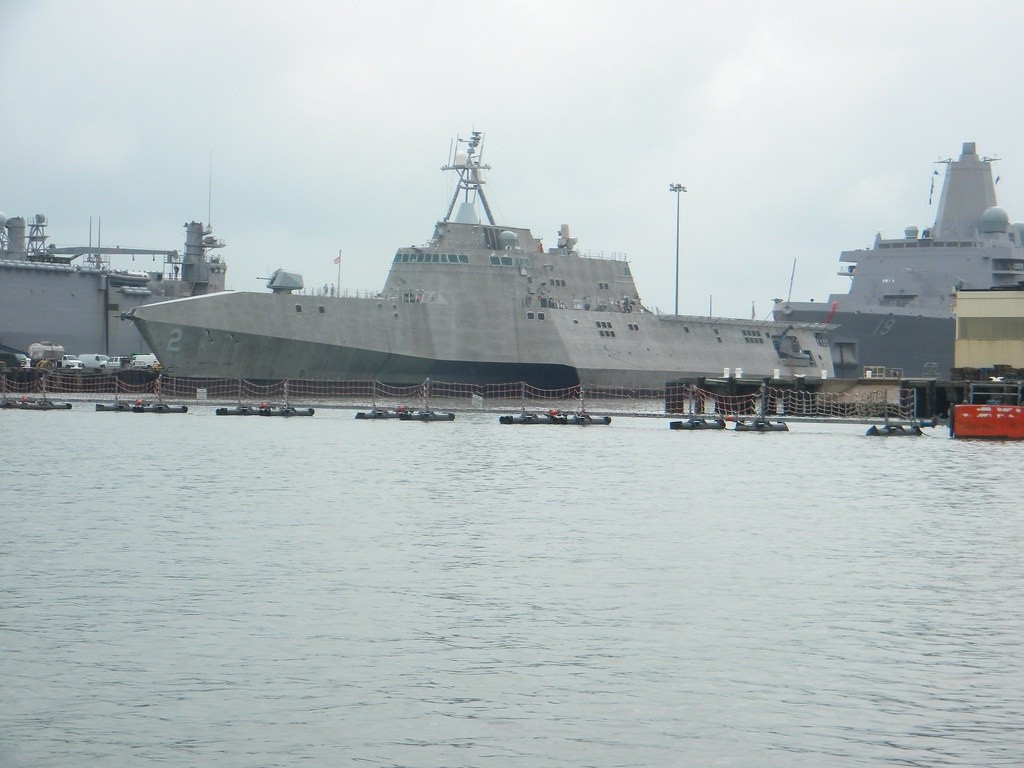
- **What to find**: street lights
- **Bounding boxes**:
[667,183,688,315]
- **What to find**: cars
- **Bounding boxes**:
[15,354,27,367]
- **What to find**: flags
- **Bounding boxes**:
[334,257,340,264]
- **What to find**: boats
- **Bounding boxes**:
[953,381,1023,441]
[96,402,133,412]
[4,397,73,410]
[113,125,845,398]
[258,404,315,417]
[0,210,234,357]
[866,425,923,438]
[133,401,188,414]
[499,412,552,424]
[735,418,789,432]
[669,417,727,430]
[355,409,406,419]
[215,405,260,416]
[770,139,1024,416]
[544,410,612,425]
[398,410,456,421]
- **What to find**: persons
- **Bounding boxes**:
[331,284,335,296]
[323,284,328,295]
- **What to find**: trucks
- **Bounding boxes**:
[131,355,159,370]
[57,355,84,370]
[79,353,110,370]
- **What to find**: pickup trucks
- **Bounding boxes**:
[98,356,146,371]
